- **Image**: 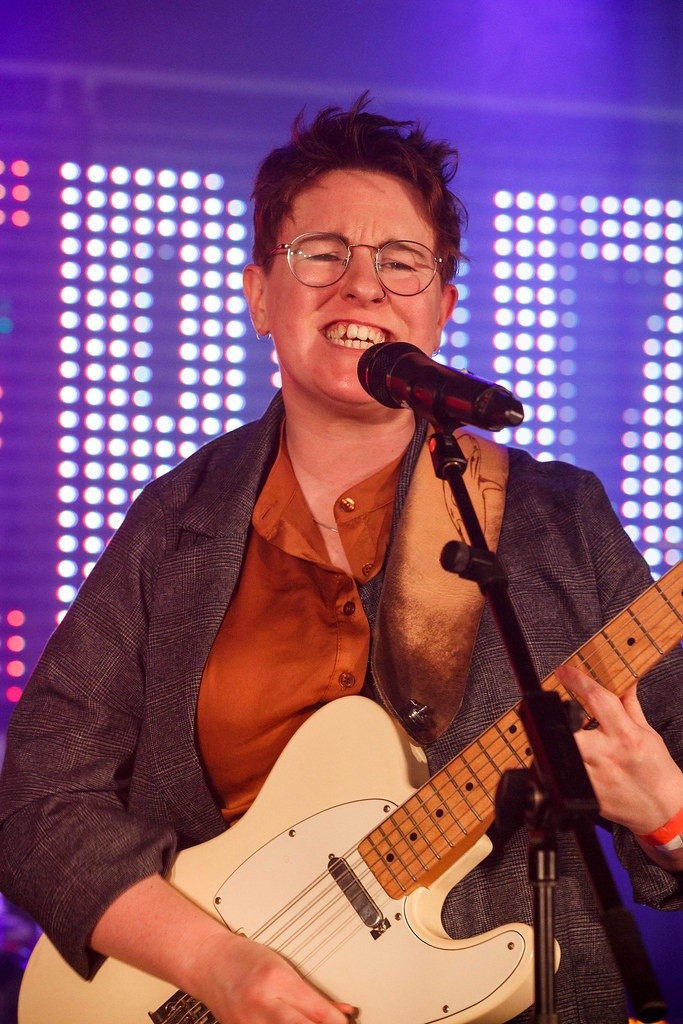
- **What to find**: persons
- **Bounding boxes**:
[0,89,683,1024]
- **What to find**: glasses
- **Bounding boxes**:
[258,232,443,296]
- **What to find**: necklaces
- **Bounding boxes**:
[312,519,338,535]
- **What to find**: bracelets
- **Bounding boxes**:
[633,808,683,873]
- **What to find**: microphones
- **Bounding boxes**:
[358,341,525,433]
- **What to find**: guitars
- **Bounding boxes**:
[17,560,683,1024]
